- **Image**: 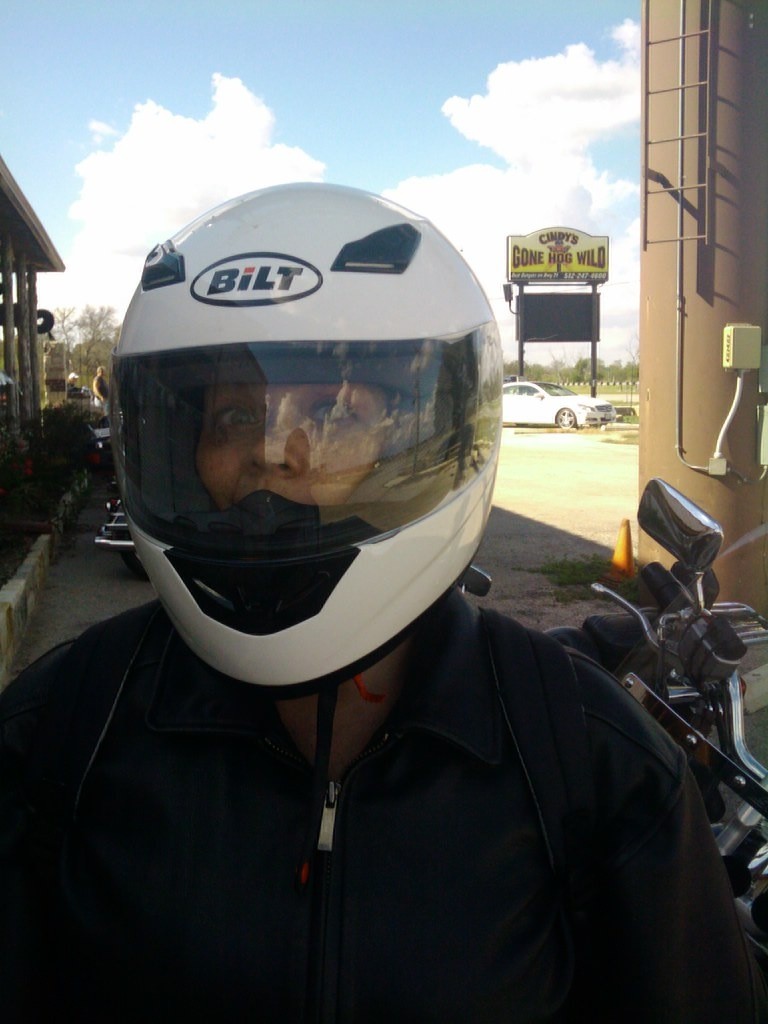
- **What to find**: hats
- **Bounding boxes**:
[68,372,79,379]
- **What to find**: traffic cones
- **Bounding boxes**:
[601,520,635,585]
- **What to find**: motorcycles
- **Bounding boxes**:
[536,479,767,976]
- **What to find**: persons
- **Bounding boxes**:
[66,372,91,399]
[0,178,746,1023]
[92,366,108,415]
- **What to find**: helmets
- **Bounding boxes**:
[108,184,502,699]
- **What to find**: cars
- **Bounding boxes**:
[500,380,618,431]
